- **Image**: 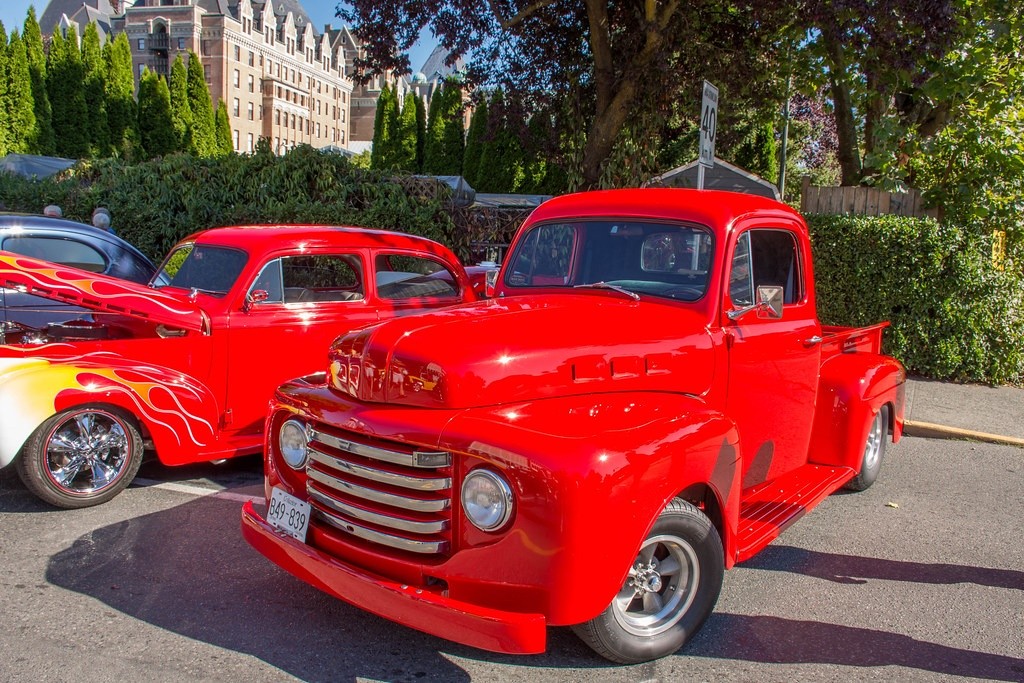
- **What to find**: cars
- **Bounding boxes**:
[0,212,157,285]
[0,224,502,509]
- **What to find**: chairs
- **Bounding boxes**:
[297,291,362,305]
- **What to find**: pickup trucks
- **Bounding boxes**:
[239,187,907,665]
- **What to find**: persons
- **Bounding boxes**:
[93,213,110,231]
[93,208,115,234]
[44,205,62,218]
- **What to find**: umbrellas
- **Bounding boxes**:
[0,153,78,182]
